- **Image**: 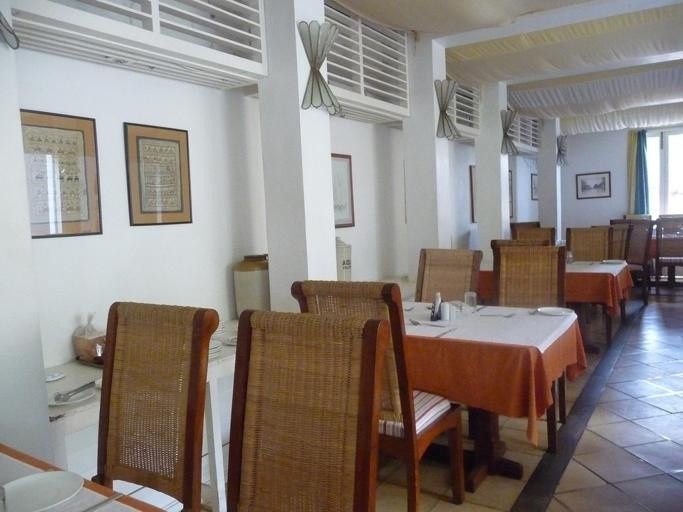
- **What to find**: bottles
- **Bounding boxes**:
[233,255,270,319]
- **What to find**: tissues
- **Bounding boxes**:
[73,312,105,361]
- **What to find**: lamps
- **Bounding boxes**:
[557,136,569,166]
[0,11,19,49]
[298,21,341,115]
[501,110,519,156]
[434,79,462,140]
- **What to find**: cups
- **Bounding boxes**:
[463,292,476,310]
[440,301,449,322]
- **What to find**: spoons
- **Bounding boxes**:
[479,313,514,318]
[409,318,445,328]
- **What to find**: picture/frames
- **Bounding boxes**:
[576,171,611,199]
[470,165,478,223]
[20,109,102,239]
[509,170,513,217]
[531,173,538,200]
[331,153,355,228]
[124,122,193,226]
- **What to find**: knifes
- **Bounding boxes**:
[435,328,457,337]
[471,306,485,314]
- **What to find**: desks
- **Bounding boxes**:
[44,317,237,512]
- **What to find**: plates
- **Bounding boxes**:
[602,259,626,264]
[1,469,84,512]
[536,307,574,316]
[207,321,237,360]
[47,386,95,406]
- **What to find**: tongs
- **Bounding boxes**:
[52,381,95,402]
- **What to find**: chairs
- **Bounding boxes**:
[226,309,390,512]
[91,300,220,512]
[291,281,464,512]
